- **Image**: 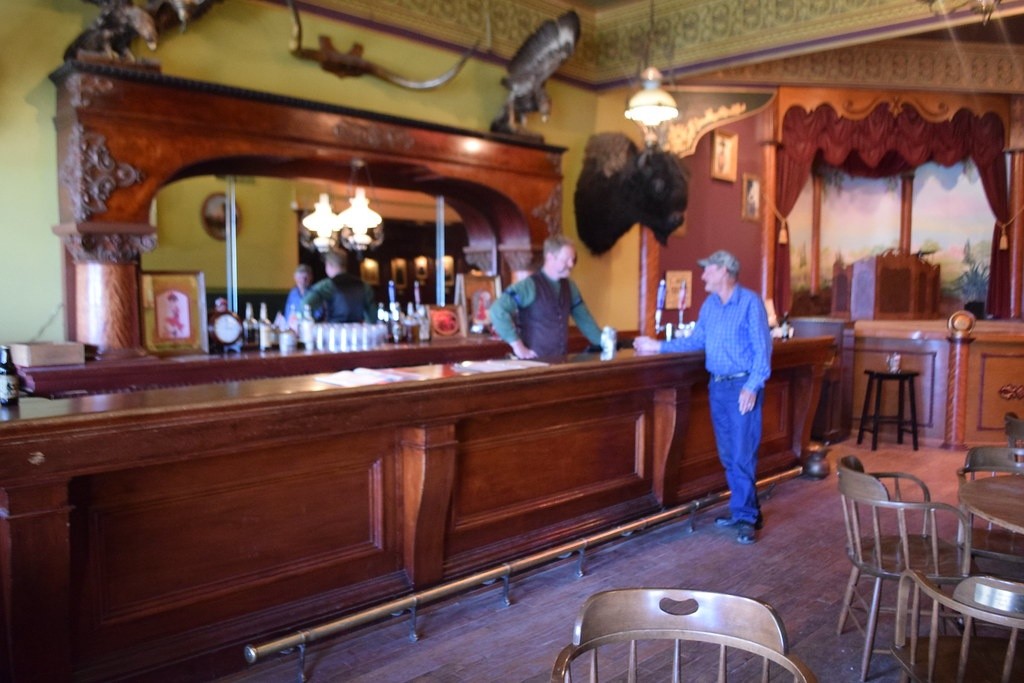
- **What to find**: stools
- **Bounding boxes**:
[857,367,921,451]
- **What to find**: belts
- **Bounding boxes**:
[712,372,750,381]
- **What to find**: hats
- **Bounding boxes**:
[697,250,739,277]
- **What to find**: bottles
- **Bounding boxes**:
[0,345,19,406]
[377,302,390,339]
[257,302,314,353]
[392,303,429,342]
[243,302,299,342]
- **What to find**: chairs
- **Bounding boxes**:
[553,589,818,683]
[835,445,1024,683]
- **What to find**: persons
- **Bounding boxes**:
[285,265,324,323]
[301,248,378,323]
[631,249,774,544]
[488,234,603,359]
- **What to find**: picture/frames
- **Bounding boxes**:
[709,127,739,182]
[201,192,242,241]
[741,173,764,224]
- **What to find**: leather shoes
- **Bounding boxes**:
[737,521,755,544]
[715,516,762,530]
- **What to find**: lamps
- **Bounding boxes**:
[296,157,382,253]
[623,0,680,127]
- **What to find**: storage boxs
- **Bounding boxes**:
[8,341,85,367]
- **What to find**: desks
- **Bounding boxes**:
[957,475,1024,638]
